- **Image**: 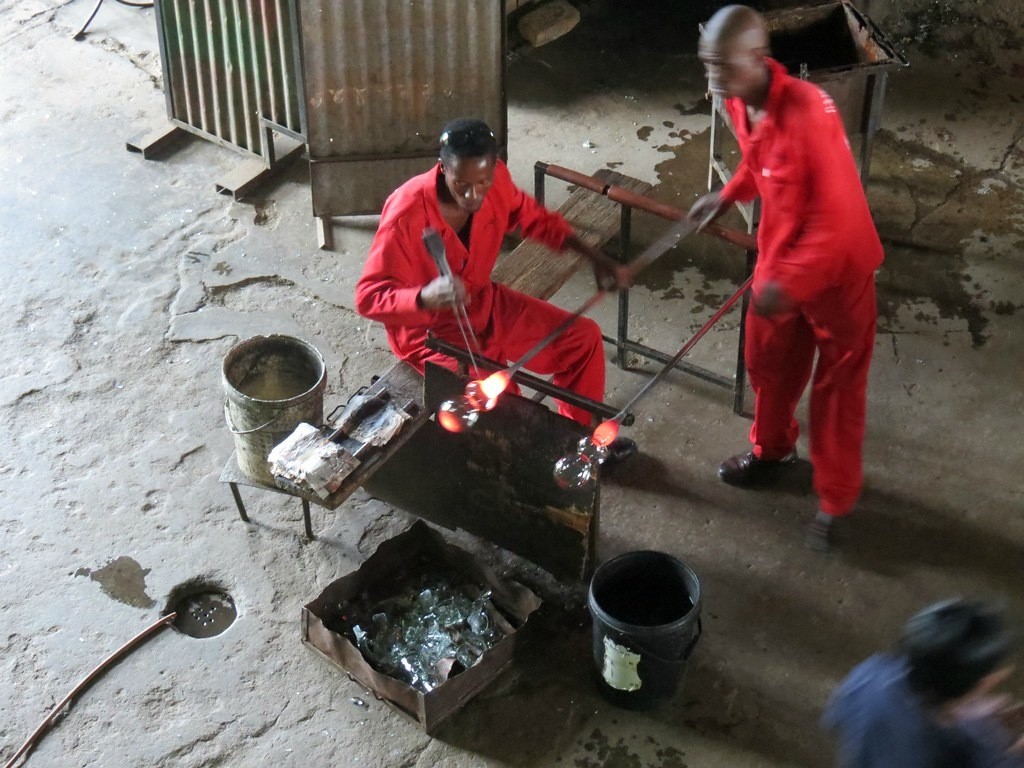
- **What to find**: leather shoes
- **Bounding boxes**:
[717,445,800,481]
[806,506,852,552]
[601,436,636,466]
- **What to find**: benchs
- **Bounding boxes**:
[273,169,652,513]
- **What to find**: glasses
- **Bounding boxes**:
[437,128,495,147]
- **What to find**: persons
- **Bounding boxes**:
[684,2,889,549]
[814,597,1024,768]
[350,117,638,464]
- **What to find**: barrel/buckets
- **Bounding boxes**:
[587,549,702,713]
[222,333,328,488]
